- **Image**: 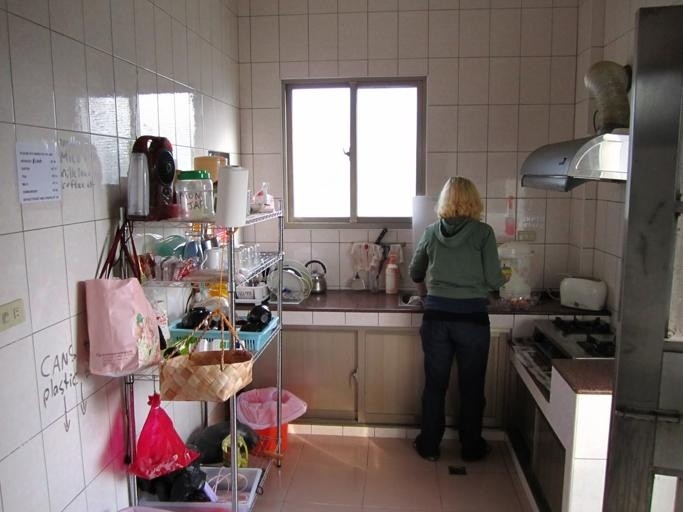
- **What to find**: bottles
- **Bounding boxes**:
[137,251,183,282]
[173,169,215,224]
[384,256,399,295]
[505,195,514,235]
[184,229,203,262]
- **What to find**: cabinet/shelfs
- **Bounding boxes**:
[252,324,508,425]
[117,210,283,512]
[509,361,677,511]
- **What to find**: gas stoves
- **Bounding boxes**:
[533,314,619,360]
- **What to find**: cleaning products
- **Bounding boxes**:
[504,194,517,236]
[384,253,400,295]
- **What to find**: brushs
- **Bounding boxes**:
[505,195,515,235]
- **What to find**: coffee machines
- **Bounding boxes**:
[128,133,176,216]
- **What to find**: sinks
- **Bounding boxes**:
[399,288,426,307]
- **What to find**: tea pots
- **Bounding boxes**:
[304,260,327,293]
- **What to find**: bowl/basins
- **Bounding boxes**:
[180,296,271,330]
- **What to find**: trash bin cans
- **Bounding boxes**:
[237,387,293,458]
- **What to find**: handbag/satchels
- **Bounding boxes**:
[84,220,162,377]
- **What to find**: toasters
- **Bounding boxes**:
[559,275,608,310]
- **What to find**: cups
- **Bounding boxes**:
[232,243,259,273]
[197,249,224,272]
[246,190,251,215]
[193,156,226,182]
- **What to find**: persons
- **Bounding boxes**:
[407,175,512,463]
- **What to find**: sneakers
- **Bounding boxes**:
[460,437,487,462]
[414,433,439,461]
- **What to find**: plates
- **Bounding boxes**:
[265,260,313,299]
